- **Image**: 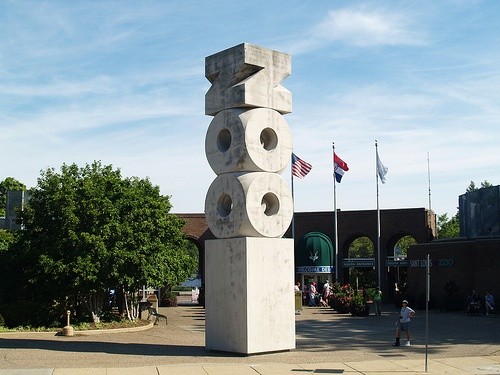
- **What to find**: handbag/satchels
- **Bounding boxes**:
[393,318,401,329]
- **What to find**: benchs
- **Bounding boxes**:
[148,307,167,326]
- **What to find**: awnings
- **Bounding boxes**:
[295,236,335,273]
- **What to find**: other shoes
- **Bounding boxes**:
[402,343,411,346]
[392,343,399,347]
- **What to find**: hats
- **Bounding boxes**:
[401,300,408,305]
[325,280,329,283]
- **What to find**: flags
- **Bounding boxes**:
[377,152,388,184]
[292,153,312,179]
[334,153,349,183]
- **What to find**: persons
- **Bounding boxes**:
[392,300,415,347]
[485,289,494,316]
[295,279,333,307]
[371,287,383,317]
[468,289,480,311]
